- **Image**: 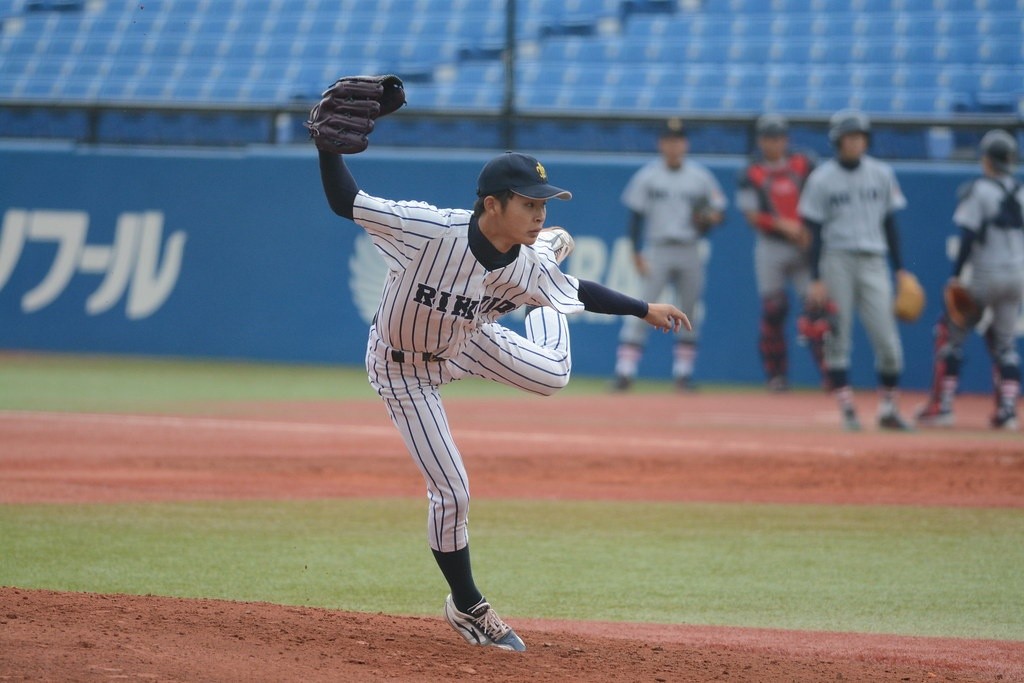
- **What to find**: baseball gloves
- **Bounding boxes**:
[943,278,986,331]
[302,73,409,157]
[894,269,927,322]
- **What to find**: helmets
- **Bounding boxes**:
[981,129,1017,154]
[828,109,872,147]
[756,112,789,134]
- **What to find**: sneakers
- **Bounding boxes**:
[445,594,527,651]
[535,226,574,268]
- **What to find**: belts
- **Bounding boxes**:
[650,238,679,246]
[392,351,446,362]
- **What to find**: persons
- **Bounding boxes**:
[306,74,692,651]
[616,119,729,395]
[735,110,835,393]
[796,107,922,434]
[914,127,1024,429]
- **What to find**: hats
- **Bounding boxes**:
[657,117,686,137]
[478,151,572,200]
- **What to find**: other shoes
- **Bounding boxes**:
[880,414,915,433]
[770,373,788,391]
[822,377,832,393]
[844,407,862,432]
[676,374,696,389]
[916,397,954,427]
[616,375,634,390]
[991,406,1018,430]
